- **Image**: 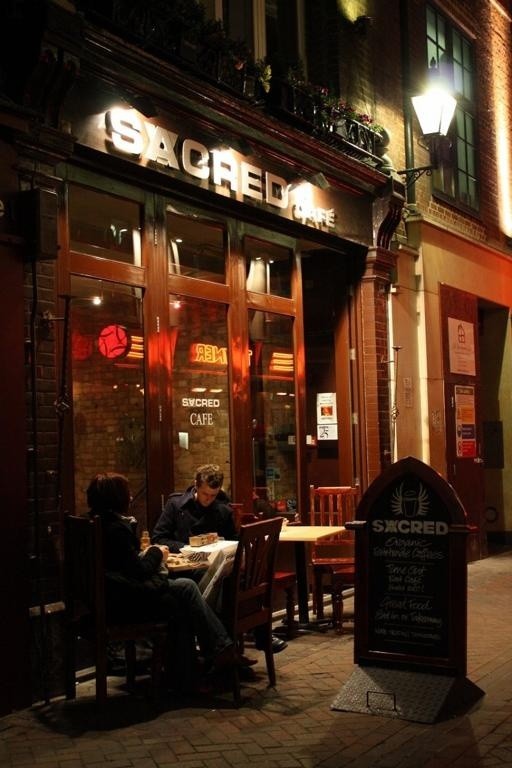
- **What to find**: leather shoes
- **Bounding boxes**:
[256,633,288,654]
[215,653,258,667]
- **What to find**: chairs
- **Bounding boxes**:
[63,510,168,728]
[209,516,283,708]
[230,504,296,638]
[161,492,184,512]
[332,564,355,635]
[306,485,361,620]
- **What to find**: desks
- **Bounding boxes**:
[265,526,346,633]
[162,540,253,578]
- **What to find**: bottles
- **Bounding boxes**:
[140,532,150,549]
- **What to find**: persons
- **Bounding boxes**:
[150,464,289,682]
[76,472,259,683]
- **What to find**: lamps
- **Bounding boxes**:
[396,57,458,190]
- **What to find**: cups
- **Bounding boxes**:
[189,536,200,547]
[208,532,217,543]
[198,534,208,545]
